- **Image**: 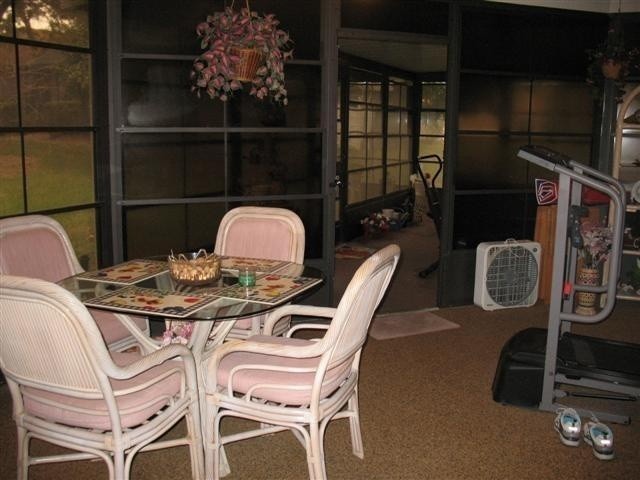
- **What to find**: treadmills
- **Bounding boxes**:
[490,144,640,426]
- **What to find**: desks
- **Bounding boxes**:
[55,253,326,480]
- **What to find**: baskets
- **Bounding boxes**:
[211,0,260,83]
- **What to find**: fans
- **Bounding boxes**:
[473,241,540,313]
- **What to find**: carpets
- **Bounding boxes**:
[368,310,460,342]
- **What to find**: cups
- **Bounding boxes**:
[237,266,257,292]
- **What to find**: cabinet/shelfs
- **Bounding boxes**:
[599,87,639,310]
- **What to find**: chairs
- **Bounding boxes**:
[172,206,306,435]
[0,214,151,355]
[199,244,401,480]
[0,275,205,480]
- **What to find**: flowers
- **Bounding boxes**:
[360,211,391,241]
[586,26,639,103]
[191,7,296,106]
[579,223,612,269]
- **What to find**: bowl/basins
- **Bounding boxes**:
[167,253,222,287]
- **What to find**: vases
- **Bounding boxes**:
[574,268,600,316]
